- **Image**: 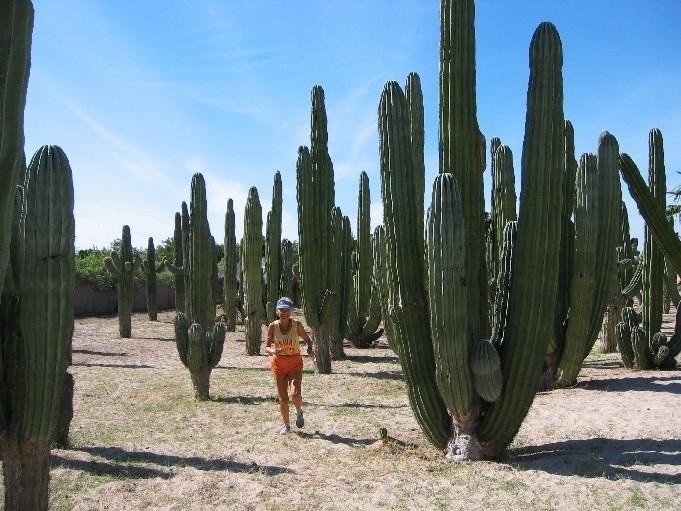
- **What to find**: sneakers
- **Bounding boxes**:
[296,410,304,428]
[281,426,290,434]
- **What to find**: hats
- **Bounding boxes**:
[277,300,293,310]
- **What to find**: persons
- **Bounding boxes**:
[263,296,315,436]
